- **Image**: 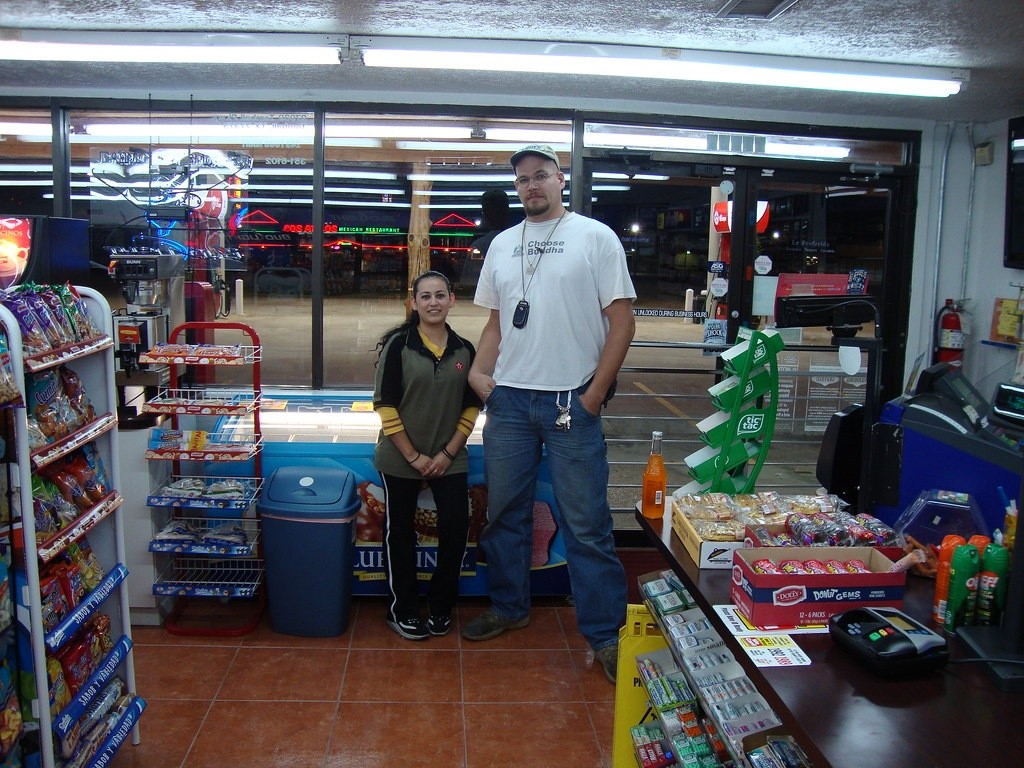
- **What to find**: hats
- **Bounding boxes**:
[509,144,561,171]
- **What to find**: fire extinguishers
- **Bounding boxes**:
[934,299,975,374]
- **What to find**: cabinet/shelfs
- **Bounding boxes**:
[136,343,267,637]
[1,286,146,768]
[689,325,780,493]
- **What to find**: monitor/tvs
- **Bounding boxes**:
[940,367,990,420]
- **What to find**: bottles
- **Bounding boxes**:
[933,535,1009,634]
[642,430,667,519]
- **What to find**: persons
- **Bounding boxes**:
[371,272,485,639]
[463,189,511,288]
[459,140,637,685]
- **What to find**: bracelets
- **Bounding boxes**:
[444,448,455,459]
[410,453,420,463]
[442,450,453,460]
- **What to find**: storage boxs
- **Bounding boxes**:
[684,330,784,495]
[730,543,908,630]
[674,494,905,570]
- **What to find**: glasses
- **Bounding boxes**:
[516,171,561,188]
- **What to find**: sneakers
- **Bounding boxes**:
[594,643,618,684]
[386,614,431,641]
[463,608,530,641]
[424,600,453,637]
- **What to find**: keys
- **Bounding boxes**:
[566,416,571,430]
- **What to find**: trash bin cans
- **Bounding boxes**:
[259,466,363,638]
[184,281,216,385]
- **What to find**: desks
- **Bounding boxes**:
[635,483,1023,765]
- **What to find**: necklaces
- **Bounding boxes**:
[520,219,557,275]
